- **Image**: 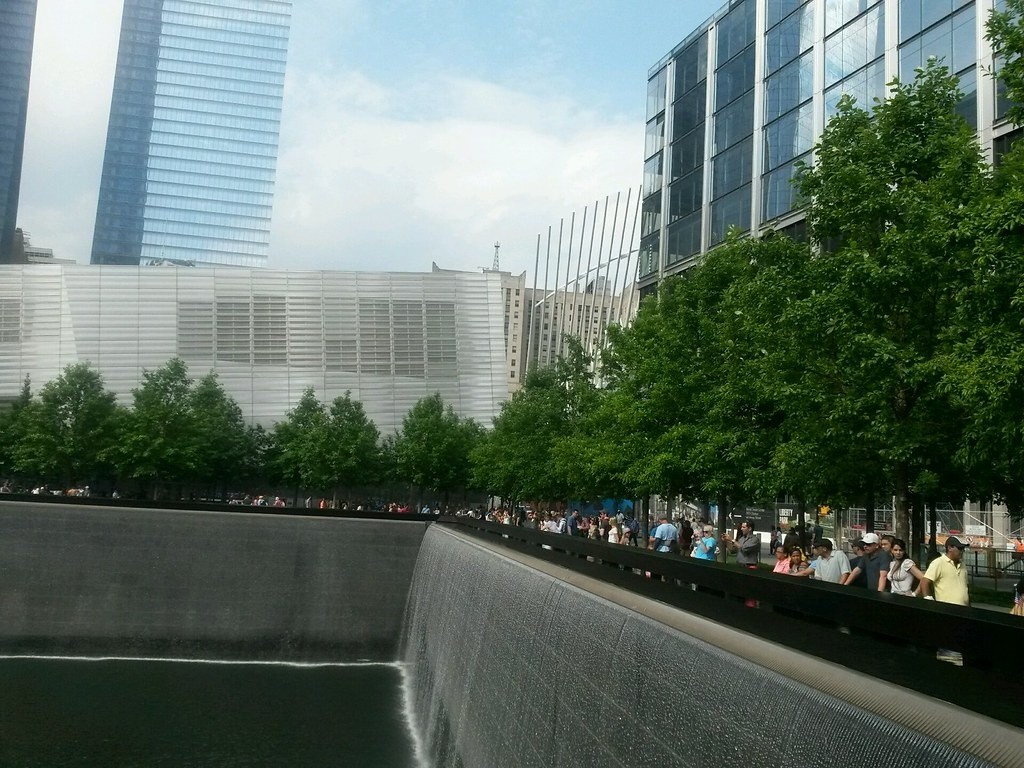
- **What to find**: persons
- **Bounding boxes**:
[455,505,512,539]
[768,519,824,560]
[434,507,440,515]
[886,539,924,598]
[512,506,641,571]
[721,517,760,607]
[228,494,286,507]
[1009,581,1024,617]
[881,536,898,561]
[632,514,717,593]
[843,532,892,593]
[421,504,431,514]
[306,497,364,511]
[444,506,453,515]
[921,536,971,669]
[382,503,410,513]
[811,540,852,585]
[1013,536,1024,553]
[772,545,819,578]
[848,536,869,589]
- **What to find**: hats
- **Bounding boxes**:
[813,539,832,550]
[659,516,668,521]
[848,536,865,551]
[623,528,630,534]
[945,537,970,548]
[600,509,610,514]
[859,533,879,544]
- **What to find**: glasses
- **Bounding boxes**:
[703,531,709,533]
[958,547,963,550]
[863,543,876,546]
[851,546,856,548]
